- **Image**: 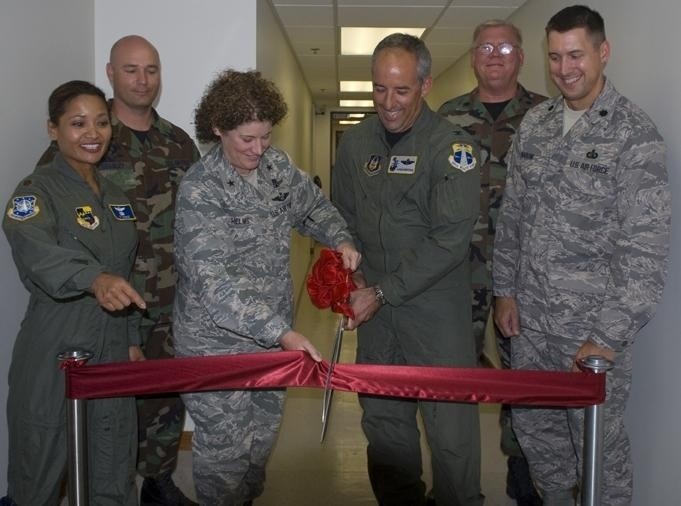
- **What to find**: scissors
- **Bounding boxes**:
[319,303,351,444]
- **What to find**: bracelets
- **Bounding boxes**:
[373,284,388,307]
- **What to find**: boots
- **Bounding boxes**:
[505,458,542,505]
[141,474,196,505]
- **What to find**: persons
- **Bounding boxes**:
[490,4,672,505]
[35,30,202,506]
[330,31,489,506]
[1,77,149,506]
[435,18,553,504]
[170,61,362,506]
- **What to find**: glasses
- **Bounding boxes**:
[475,44,515,56]
